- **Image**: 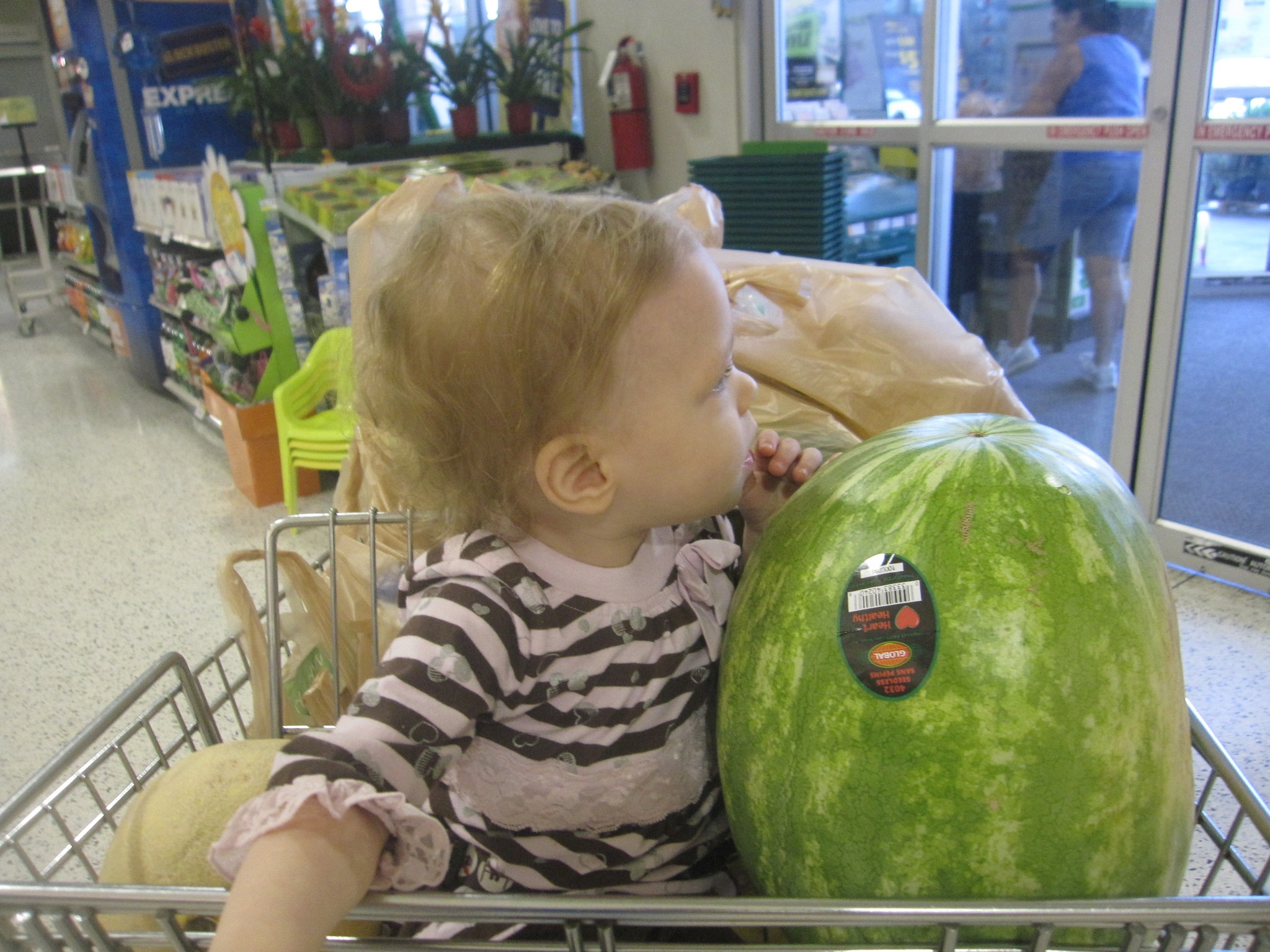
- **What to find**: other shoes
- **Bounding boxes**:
[992,336,1041,377]
[1074,351,1119,391]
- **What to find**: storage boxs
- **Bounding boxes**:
[202,389,322,511]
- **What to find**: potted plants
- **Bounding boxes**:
[219,13,598,148]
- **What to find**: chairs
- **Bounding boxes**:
[274,326,365,538]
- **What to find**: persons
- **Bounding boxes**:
[206,193,846,952]
[994,0,1151,391]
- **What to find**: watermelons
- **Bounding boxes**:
[719,414,1198,944]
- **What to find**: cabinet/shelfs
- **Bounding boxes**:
[39,49,114,350]
[133,223,265,444]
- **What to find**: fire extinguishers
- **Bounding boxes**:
[605,35,654,170]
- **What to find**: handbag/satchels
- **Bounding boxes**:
[675,179,1038,464]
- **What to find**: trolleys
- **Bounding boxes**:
[0,503,1270,952]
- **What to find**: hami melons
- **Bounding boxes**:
[86,738,379,944]
